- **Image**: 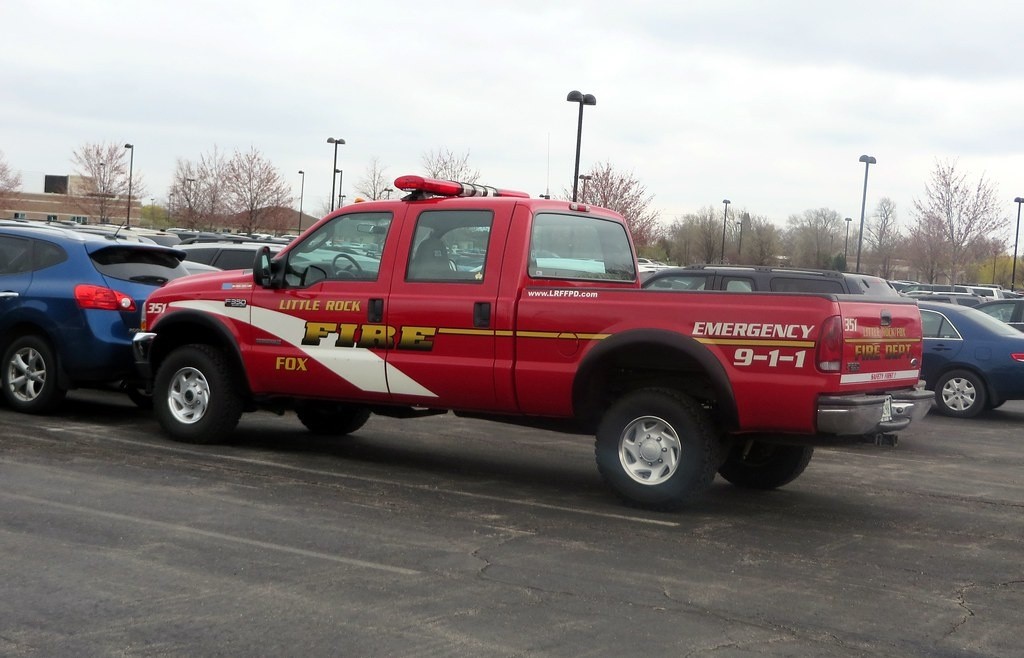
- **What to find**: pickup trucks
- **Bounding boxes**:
[128,174,936,512]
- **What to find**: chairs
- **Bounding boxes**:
[415,236,448,274]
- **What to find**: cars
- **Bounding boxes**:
[0,216,1024,420]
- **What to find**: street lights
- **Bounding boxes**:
[100,162,106,222]
[1010,197,1024,291]
[854,154,877,273]
[843,217,853,271]
[335,168,343,209]
[183,177,197,230]
[736,221,743,264]
[566,90,597,202]
[384,187,393,199]
[326,137,346,212]
[579,174,592,204]
[339,195,346,208]
[123,143,133,227]
[721,199,731,264]
[298,170,305,235]
